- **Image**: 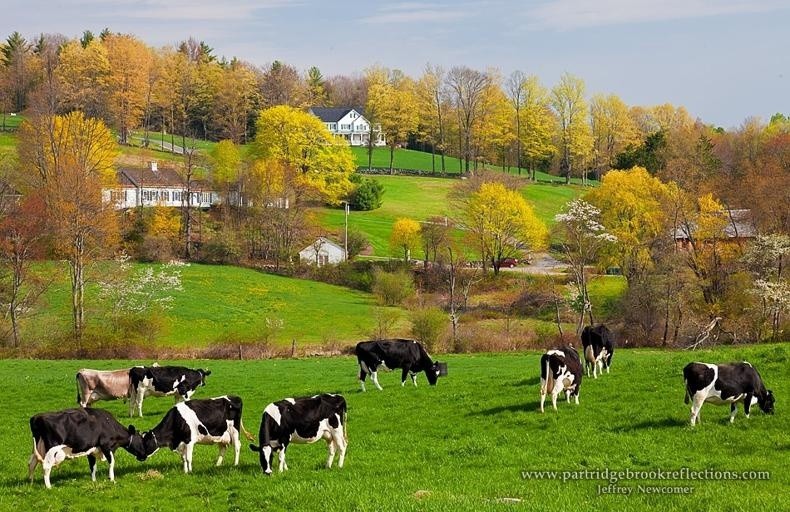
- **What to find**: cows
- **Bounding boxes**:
[683,361,776,428]
[581,324,615,379]
[355,338,442,393]
[27,408,147,490]
[76,362,213,417]
[249,392,349,476]
[137,394,256,475]
[539,346,583,413]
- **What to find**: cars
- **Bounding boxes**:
[490,256,518,268]
